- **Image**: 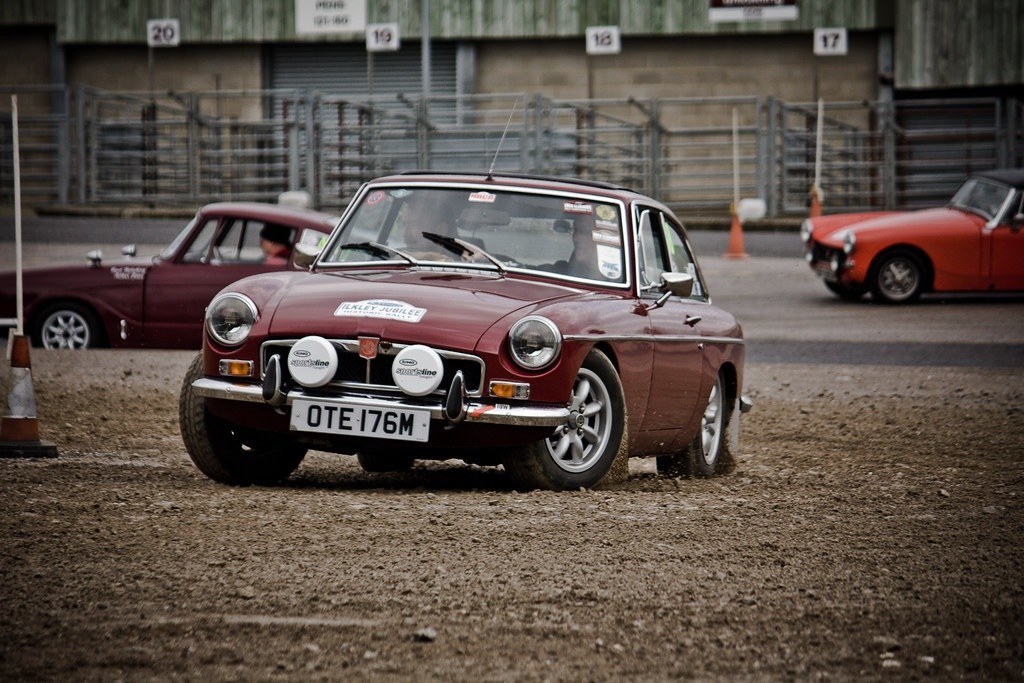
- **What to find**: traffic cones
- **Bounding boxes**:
[721,215,750,261]
[1,335,61,459]
[808,192,822,218]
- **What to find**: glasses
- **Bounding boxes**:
[565,226,593,237]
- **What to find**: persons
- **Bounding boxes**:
[402,192,460,255]
[521,214,610,280]
[210,220,292,265]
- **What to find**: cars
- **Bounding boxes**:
[0,198,378,346]
[177,168,755,490]
[799,166,1022,303]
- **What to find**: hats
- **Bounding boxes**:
[260,223,293,249]
